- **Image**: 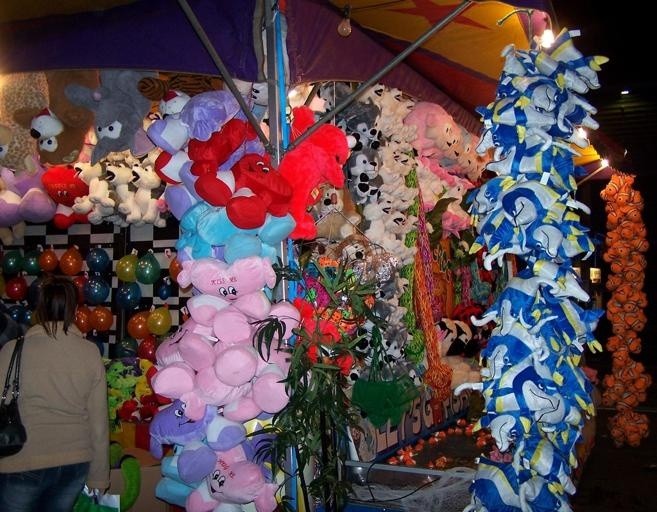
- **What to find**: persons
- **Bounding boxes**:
[0,277,112,512]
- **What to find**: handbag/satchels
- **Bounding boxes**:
[73,483,121,512]
[0,334,26,458]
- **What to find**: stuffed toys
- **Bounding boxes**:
[600,174,652,448]
[465,28,611,512]
[0,7,652,512]
[105,73,349,512]
[0,69,169,228]
[312,80,490,430]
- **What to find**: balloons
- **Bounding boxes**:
[1,246,183,363]
